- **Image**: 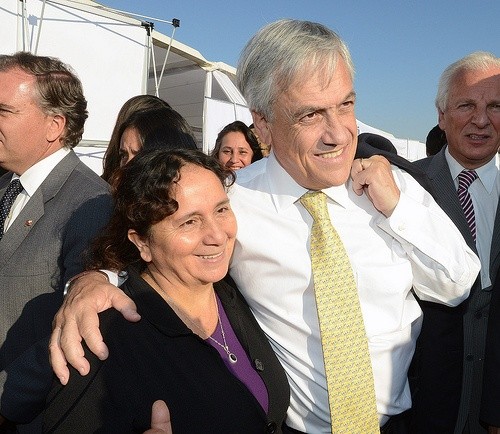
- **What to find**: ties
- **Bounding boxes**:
[300,191,381,434]
[456,169,479,246]
[0,179,23,240]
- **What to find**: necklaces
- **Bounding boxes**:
[146,269,239,364]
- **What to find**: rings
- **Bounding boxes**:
[359,158,364,172]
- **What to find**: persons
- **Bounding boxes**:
[98,54,449,186]
[18,147,290,434]
[49,18,481,434]
[410,50,500,434]
[0,50,114,424]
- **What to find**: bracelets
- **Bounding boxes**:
[62,270,110,297]
